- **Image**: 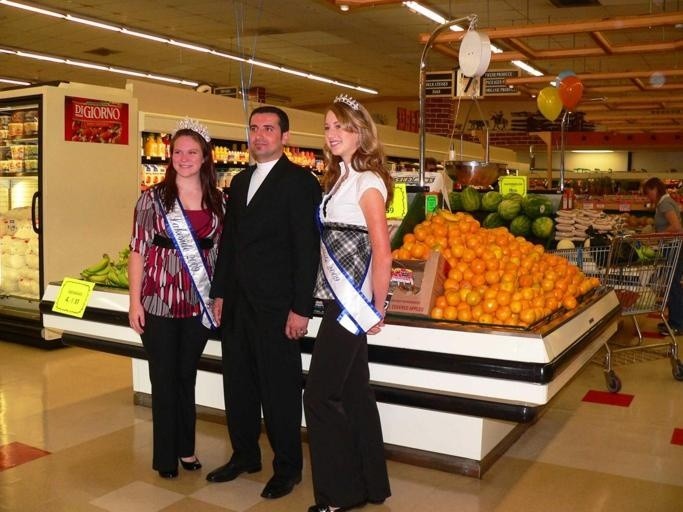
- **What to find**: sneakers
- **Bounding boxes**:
[658,320,683,336]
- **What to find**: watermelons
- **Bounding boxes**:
[449,187,554,236]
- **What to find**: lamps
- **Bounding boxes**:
[397,2,559,89]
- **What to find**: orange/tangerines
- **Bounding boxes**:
[391,211,600,329]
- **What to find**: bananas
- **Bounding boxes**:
[80,247,129,288]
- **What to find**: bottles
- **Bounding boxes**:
[140,134,316,188]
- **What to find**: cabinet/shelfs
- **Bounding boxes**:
[142,130,418,193]
[535,170,680,233]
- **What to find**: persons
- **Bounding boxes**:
[426,158,438,172]
[127,115,227,480]
[71,120,123,144]
[302,92,393,511]
[644,177,683,336]
[435,161,444,169]
[209,105,322,500]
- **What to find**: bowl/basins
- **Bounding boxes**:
[444,160,505,187]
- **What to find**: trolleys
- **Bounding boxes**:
[545,231,682,394]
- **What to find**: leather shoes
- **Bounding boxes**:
[367,497,385,504]
[308,494,366,512]
[180,456,202,471]
[206,454,262,483]
[158,468,179,478]
[260,471,302,499]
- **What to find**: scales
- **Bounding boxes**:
[445,30,508,186]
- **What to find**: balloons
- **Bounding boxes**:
[537,86,562,123]
[557,68,576,89]
[559,76,584,108]
